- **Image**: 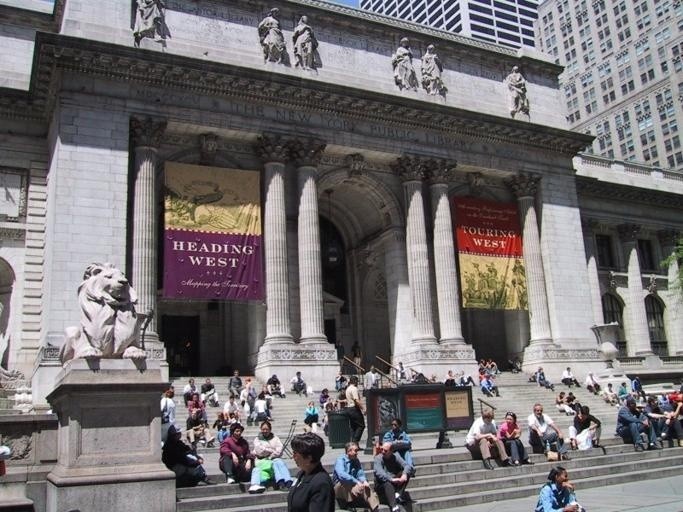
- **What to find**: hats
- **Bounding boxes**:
[168,425,183,434]
[627,393,683,403]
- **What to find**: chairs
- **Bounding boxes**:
[280,420,298,459]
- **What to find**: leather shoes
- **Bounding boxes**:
[348,497,407,512]
[484,450,571,470]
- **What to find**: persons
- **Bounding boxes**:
[219,422,266,494]
[332,441,380,512]
[287,432,336,512]
[374,442,410,511]
[535,467,586,512]
[292,15,323,72]
[162,425,217,487]
[131,0,172,42]
[421,44,448,101]
[160,367,366,448]
[258,7,291,67]
[528,366,683,461]
[391,37,419,92]
[253,421,293,490]
[503,65,530,121]
[381,418,418,478]
[338,341,535,469]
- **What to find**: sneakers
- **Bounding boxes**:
[192,442,293,494]
[635,437,683,452]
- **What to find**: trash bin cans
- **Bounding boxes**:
[325,410,351,449]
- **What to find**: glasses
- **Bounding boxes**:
[392,424,396,426]
[537,410,542,412]
[381,448,387,452]
[507,412,513,415]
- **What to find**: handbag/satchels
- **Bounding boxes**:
[575,428,594,450]
[161,407,170,424]
